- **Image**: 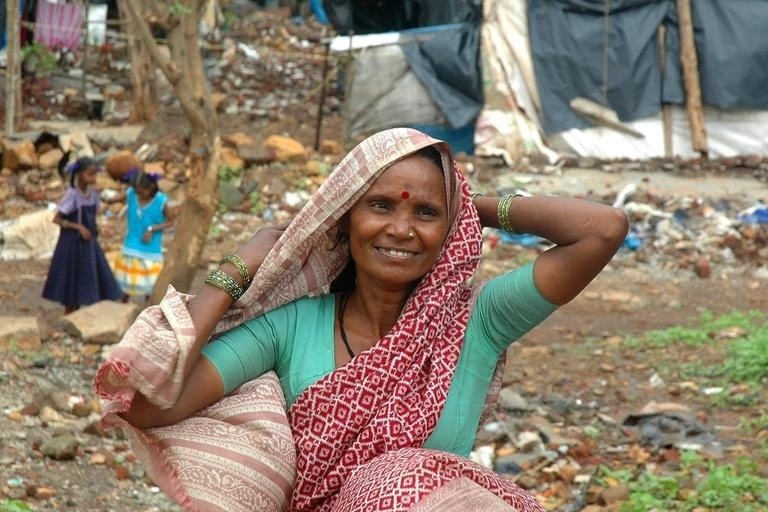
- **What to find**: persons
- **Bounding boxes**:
[100,166,177,305]
[42,157,125,315]
[113,127,631,510]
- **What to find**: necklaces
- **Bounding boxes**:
[334,286,371,366]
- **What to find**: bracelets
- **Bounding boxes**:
[470,191,481,230]
[205,269,244,302]
[496,194,525,236]
[219,254,249,290]
[146,225,152,232]
